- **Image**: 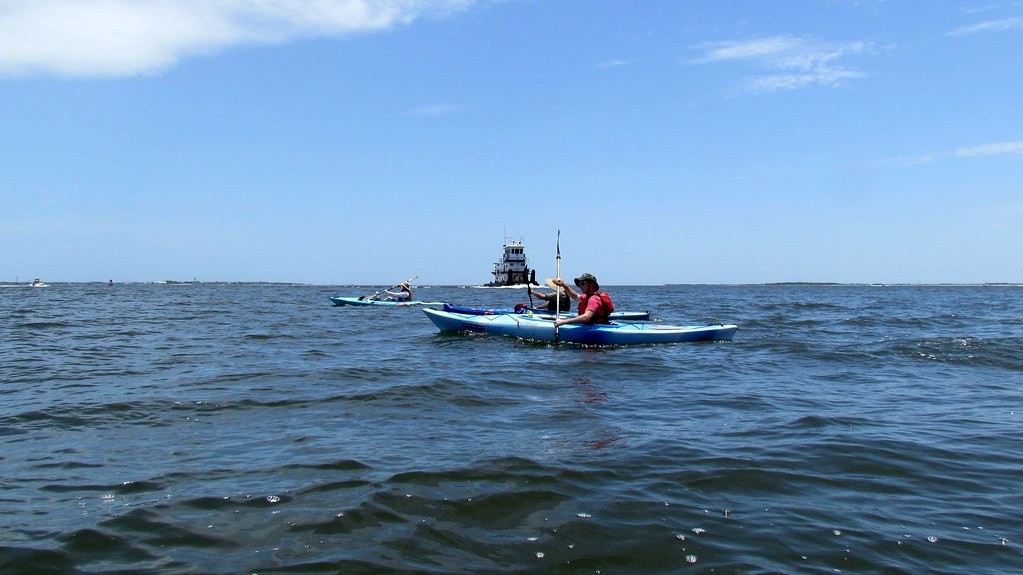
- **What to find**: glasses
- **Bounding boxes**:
[579,282,586,285]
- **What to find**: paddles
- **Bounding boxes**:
[367,275,420,300]
[555,229,561,348]
[527,282,533,309]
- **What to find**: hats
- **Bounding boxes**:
[401,282,411,290]
[574,273,599,291]
[546,278,568,292]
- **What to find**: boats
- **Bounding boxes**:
[329,296,449,306]
[483,227,539,288]
[33,279,42,286]
[423,307,737,344]
[443,304,650,323]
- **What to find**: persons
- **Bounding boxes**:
[552,273,614,326]
[527,276,571,312]
[384,282,412,301]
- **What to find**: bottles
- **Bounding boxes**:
[528,311,533,318]
